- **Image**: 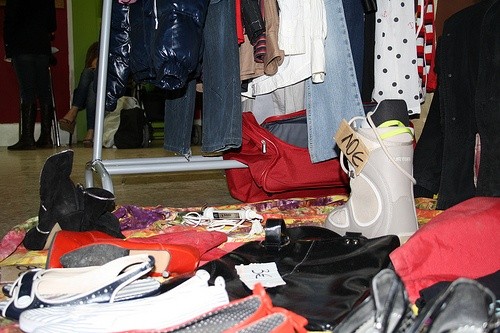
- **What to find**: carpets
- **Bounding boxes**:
[0,194,445,332]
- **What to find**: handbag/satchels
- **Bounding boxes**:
[225,108,350,201]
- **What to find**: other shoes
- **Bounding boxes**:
[6,117,74,151]
[324,100,419,235]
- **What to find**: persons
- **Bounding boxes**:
[59,41,100,148]
[3,0,58,150]
